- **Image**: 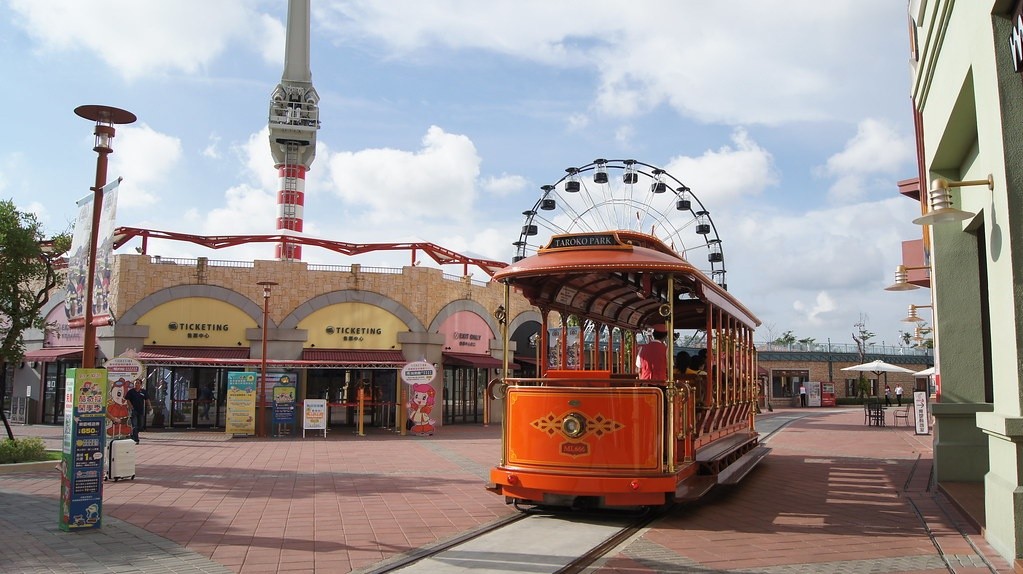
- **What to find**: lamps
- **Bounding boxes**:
[30,362,37,368]
[901,303,934,322]
[884,264,931,292]
[908,326,934,340]
[433,363,438,368]
[496,367,500,375]
[911,174,994,225]
[107,318,116,327]
[469,273,474,278]
[913,337,933,349]
[18,362,24,369]
[414,260,421,266]
[135,246,144,253]
[101,358,107,363]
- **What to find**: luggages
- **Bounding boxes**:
[104,421,135,481]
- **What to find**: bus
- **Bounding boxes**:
[485,227,773,511]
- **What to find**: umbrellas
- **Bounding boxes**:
[840,360,916,426]
[913,367,934,378]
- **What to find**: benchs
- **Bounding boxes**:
[610,375,709,409]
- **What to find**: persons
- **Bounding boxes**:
[885,385,891,407]
[893,383,904,406]
[200,383,216,421]
[799,384,806,407]
[635,324,668,383]
[674,348,730,385]
[125,379,152,445]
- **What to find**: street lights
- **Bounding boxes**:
[255,281,280,437]
[73,104,138,371]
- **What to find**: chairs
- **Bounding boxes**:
[893,402,910,427]
[864,401,885,427]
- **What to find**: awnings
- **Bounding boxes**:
[25,349,83,362]
[443,353,521,369]
[304,350,406,362]
[137,348,250,359]
[515,357,540,369]
[742,363,768,375]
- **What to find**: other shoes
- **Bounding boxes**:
[135,441,138,445]
